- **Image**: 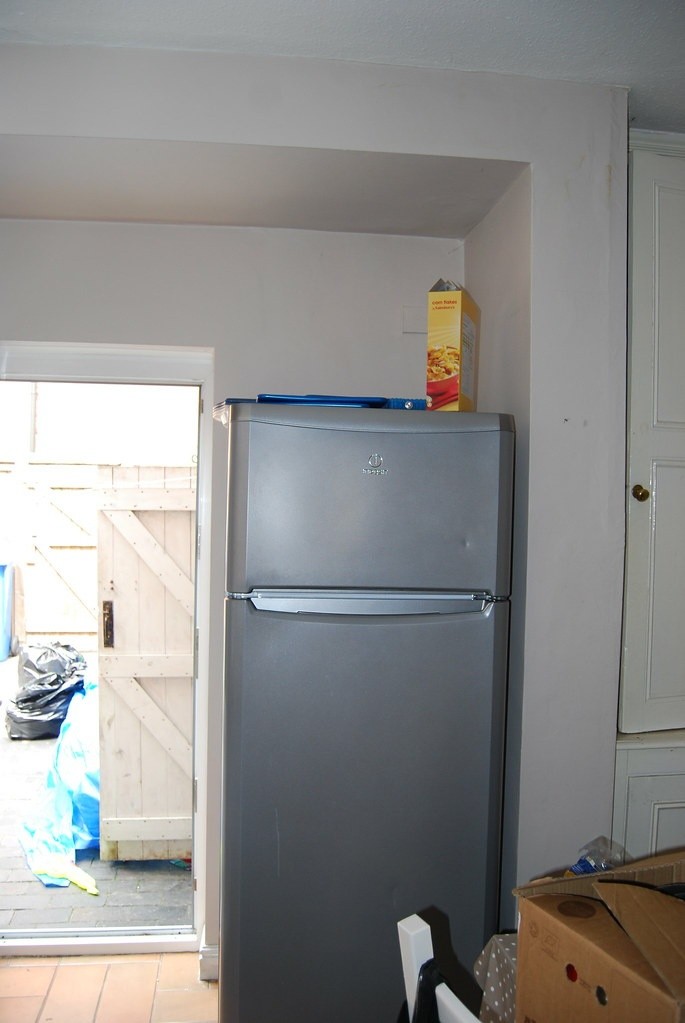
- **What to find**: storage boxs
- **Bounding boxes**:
[512,847,685,1023]
[422,275,485,410]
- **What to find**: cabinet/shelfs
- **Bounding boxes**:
[614,128,685,856]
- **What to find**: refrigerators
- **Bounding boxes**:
[217,402,516,1023]
